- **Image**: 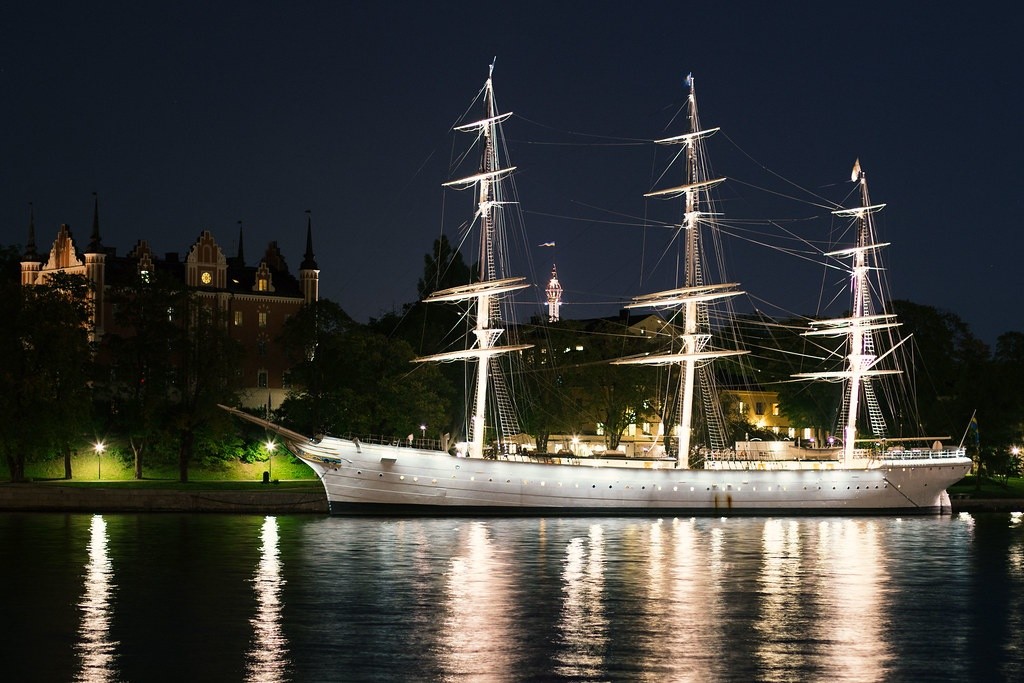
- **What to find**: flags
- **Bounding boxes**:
[967,412,980,450]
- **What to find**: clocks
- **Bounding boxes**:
[202,273,212,284]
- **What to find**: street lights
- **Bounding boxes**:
[420,425,426,438]
[269,443,272,478]
[97,445,101,479]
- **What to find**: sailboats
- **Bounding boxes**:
[214,55,973,518]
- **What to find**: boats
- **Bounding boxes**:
[950,493,1001,511]
